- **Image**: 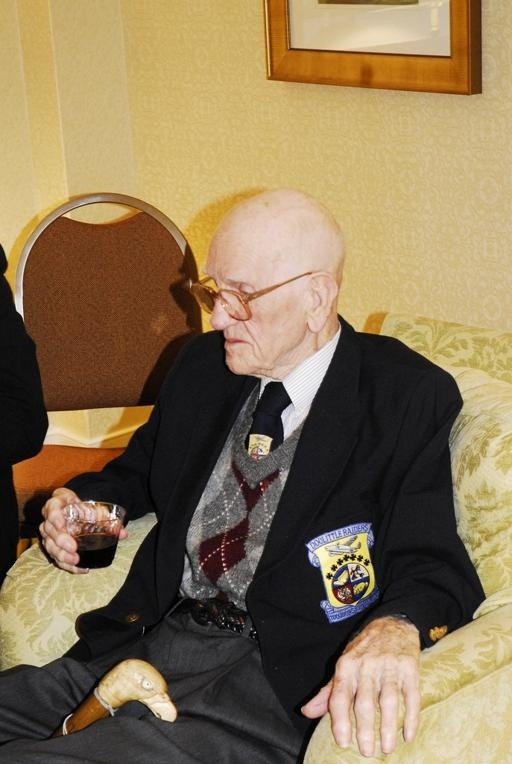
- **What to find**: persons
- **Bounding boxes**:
[0,242,49,590]
[0,188,485,764]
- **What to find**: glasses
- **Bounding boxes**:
[190,271,314,322]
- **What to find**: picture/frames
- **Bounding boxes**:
[264,0,481,95]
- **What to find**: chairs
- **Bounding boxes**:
[3,305,510,763]
[12,187,203,418]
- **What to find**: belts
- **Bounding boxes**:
[176,597,259,640]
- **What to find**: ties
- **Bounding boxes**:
[244,381,293,459]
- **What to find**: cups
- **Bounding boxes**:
[60,502,126,569]
